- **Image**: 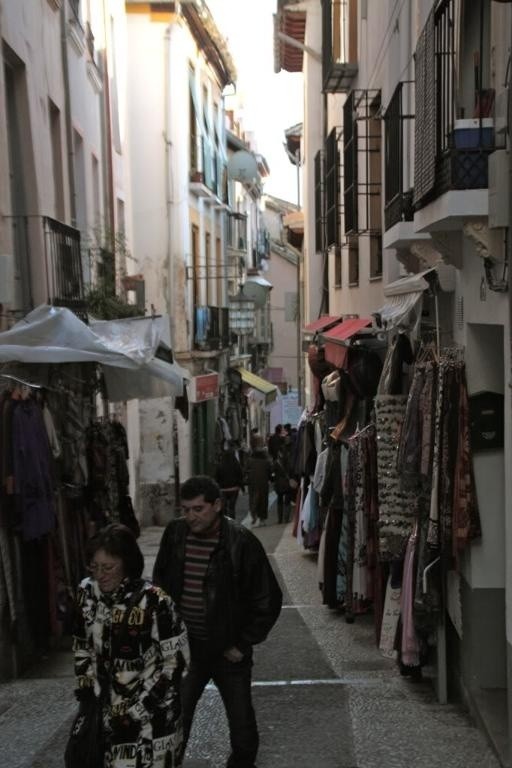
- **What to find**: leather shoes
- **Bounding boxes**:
[284,520,291,523]
[278,519,283,524]
[259,522,267,527]
[252,520,256,524]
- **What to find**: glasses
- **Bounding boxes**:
[85,561,123,574]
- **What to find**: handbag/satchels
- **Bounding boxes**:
[64,700,107,767]
[276,460,297,489]
[259,465,270,490]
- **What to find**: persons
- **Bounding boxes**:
[241,446,275,526]
[150,474,283,768]
[269,423,286,460]
[264,433,272,448]
[71,523,190,768]
[273,445,298,524]
[282,422,292,435]
[249,427,264,448]
[210,439,246,519]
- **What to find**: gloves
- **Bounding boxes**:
[223,646,244,663]
